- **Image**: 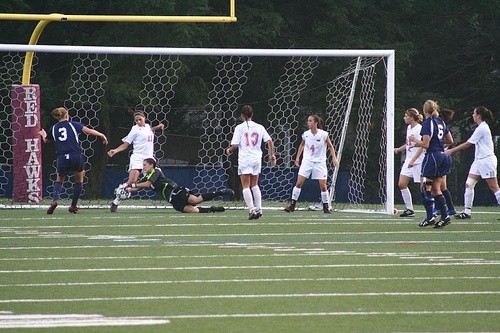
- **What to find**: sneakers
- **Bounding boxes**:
[455,212,471,219]
[431,208,441,217]
[220,188,235,196]
[323,208,331,213]
[68,206,79,214]
[109,200,121,212]
[399,209,416,217]
[284,205,295,212]
[210,206,226,213]
[419,217,435,227]
[248,209,262,220]
[46,200,58,214]
[446,209,457,216]
[433,215,452,229]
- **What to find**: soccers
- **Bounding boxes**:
[116,186,131,200]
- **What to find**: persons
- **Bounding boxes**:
[445,107,500,221]
[107,111,164,213]
[227,105,276,220]
[407,135,457,216]
[409,100,453,229]
[38,107,108,215]
[115,158,234,214]
[395,108,427,216]
[285,115,338,214]
